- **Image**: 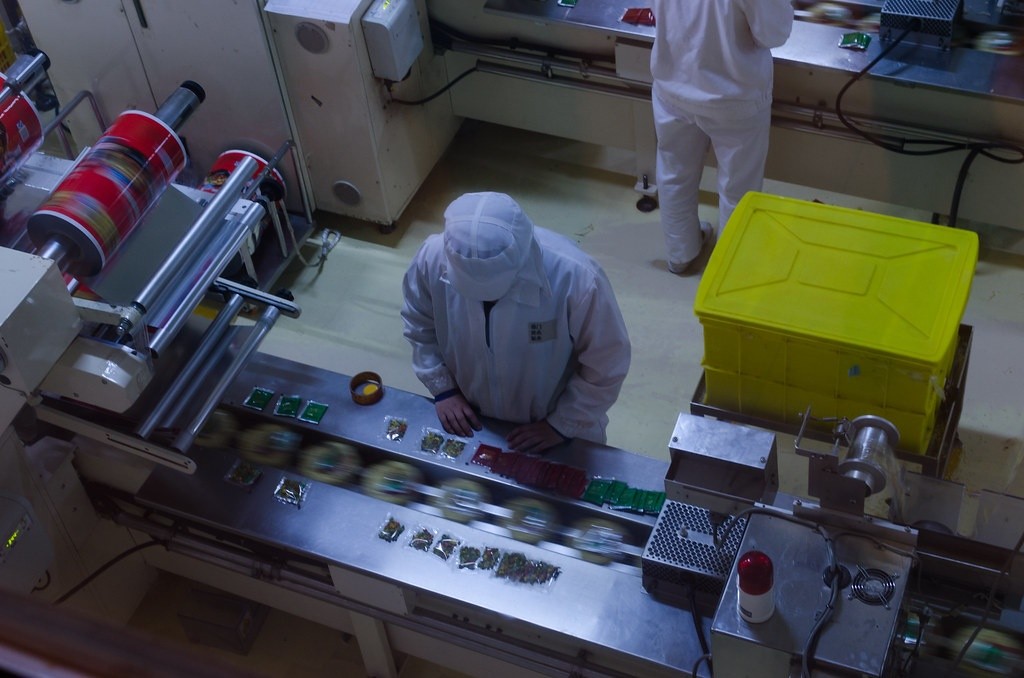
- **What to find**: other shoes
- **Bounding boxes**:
[668,221,713,273]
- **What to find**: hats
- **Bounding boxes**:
[443,192,552,308]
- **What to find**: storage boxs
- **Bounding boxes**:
[178,588,267,657]
[695,190,980,455]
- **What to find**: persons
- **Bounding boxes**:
[649,0,794,275]
[400,192,632,455]
